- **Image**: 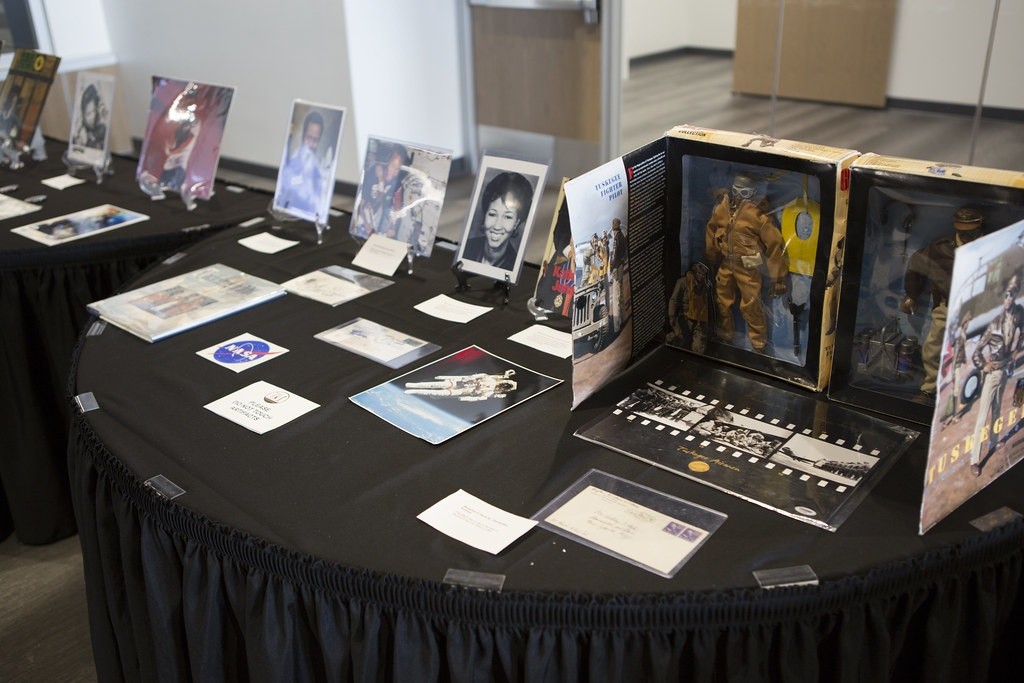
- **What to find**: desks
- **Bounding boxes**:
[65,212,1024,682]
[0,136,275,545]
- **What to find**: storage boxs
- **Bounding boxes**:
[827,152,1024,428]
[664,124,862,393]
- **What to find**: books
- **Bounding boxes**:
[85,260,289,344]
[9,203,152,248]
[571,359,909,533]
[0,47,62,154]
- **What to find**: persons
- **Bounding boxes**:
[900,204,992,402]
[463,170,534,272]
[938,273,1024,477]
[404,372,519,403]
[586,216,628,338]
[277,110,326,216]
[705,170,790,357]
[76,83,107,151]
[159,91,203,191]
[668,260,717,359]
[354,143,411,238]
